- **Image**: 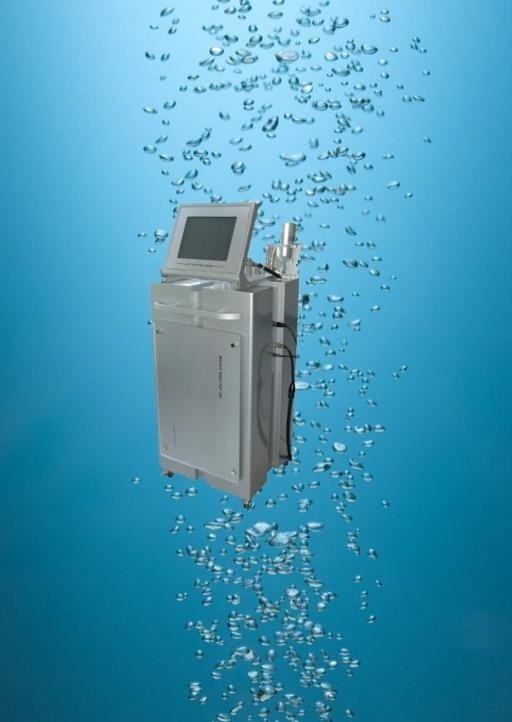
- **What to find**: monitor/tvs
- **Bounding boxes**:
[162,200,257,282]
